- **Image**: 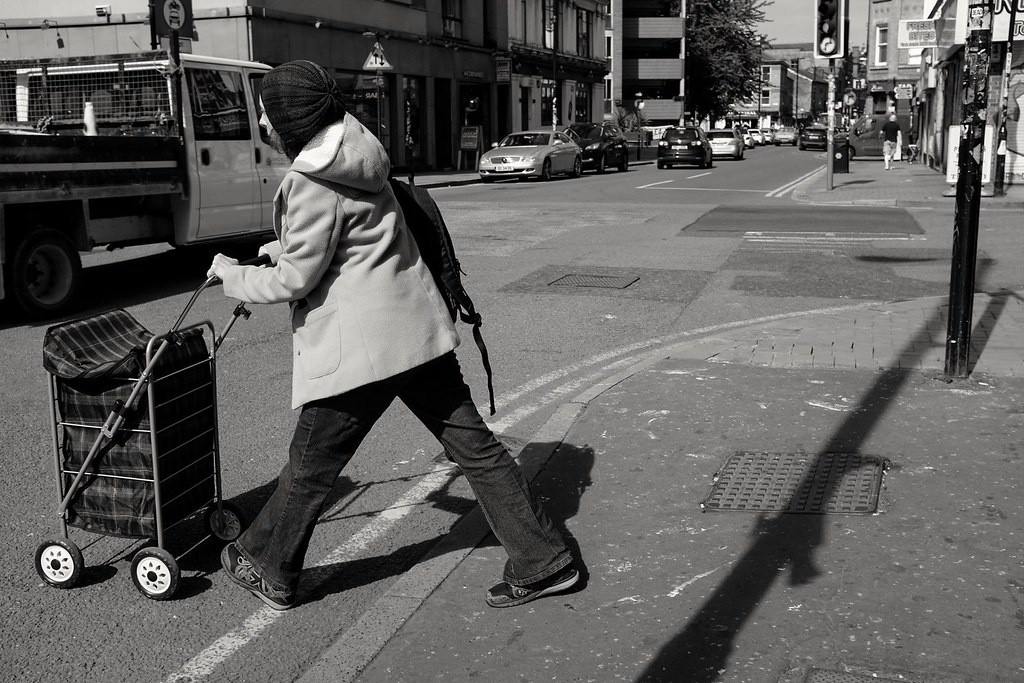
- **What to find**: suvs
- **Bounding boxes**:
[563,121,630,175]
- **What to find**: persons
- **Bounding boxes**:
[207,62,580,609]
[879,114,903,170]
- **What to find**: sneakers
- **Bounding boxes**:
[220,542,296,610]
[485,562,579,608]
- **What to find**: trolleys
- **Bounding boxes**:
[35,254,272,602]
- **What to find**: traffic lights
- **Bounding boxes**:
[814,0,844,59]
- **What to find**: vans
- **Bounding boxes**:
[834,114,917,160]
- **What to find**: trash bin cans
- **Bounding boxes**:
[833,139,849,173]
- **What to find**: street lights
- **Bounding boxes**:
[758,37,769,119]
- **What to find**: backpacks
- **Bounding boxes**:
[389,177,477,323]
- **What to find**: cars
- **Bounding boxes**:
[761,128,774,144]
[799,126,827,151]
[774,127,799,146]
[705,129,745,161]
[749,129,766,146]
[478,130,583,183]
[740,130,756,149]
[657,126,713,169]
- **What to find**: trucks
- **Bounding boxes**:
[0,52,291,326]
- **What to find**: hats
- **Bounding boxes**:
[260,59,343,154]
